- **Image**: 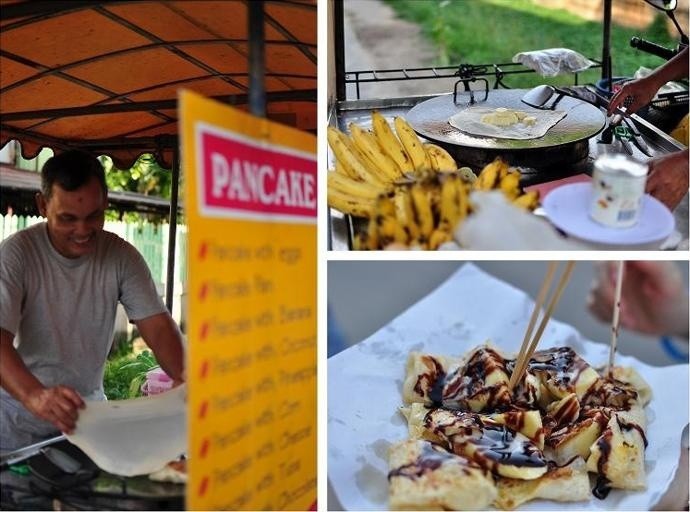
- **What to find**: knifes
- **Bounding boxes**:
[617,111,652,157]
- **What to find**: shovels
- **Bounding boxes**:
[522,85,600,108]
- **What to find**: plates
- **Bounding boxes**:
[546,180,675,245]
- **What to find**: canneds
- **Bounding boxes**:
[588,158,649,229]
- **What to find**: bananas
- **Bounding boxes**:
[327,109,540,251]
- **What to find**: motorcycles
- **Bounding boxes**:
[630,0,689,61]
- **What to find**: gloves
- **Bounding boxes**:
[595,76,689,133]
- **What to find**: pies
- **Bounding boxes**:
[63,382,188,477]
[388,344,652,511]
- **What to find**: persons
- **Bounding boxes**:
[588,261,689,335]
[607,45,689,213]
[0,154,184,456]
